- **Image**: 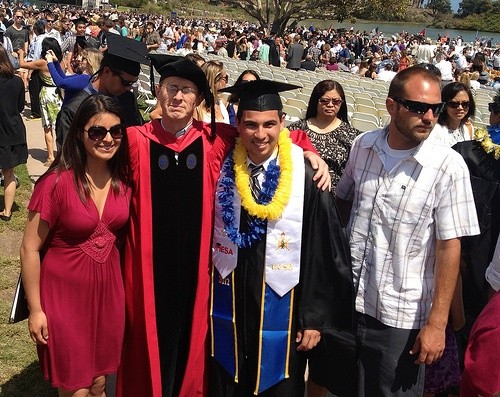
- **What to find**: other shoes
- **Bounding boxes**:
[0,214,12,221]
[29,114,37,119]
[43,158,55,166]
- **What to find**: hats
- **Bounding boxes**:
[149,53,215,141]
[71,17,89,25]
[103,32,156,98]
[217,78,304,112]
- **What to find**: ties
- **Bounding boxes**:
[249,163,265,203]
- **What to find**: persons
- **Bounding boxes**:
[0,41,29,219]
[227,69,259,126]
[15,37,62,167]
[145,23,160,50]
[19,94,132,397]
[147,53,207,119]
[194,58,230,125]
[45,47,104,150]
[5,12,29,92]
[284,79,362,228]
[55,30,148,164]
[433,82,476,149]
[61,16,97,52]
[29,19,49,64]
[334,65,483,397]
[124,53,333,397]
[0,1,500,397]
[208,80,355,397]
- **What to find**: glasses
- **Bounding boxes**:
[488,103,496,112]
[47,20,54,23]
[160,85,200,97]
[391,96,446,115]
[447,101,471,108]
[82,123,125,141]
[16,16,23,19]
[319,98,342,106]
[116,74,137,86]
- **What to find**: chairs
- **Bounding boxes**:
[133,48,500,141]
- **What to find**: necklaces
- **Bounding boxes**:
[219,149,281,247]
[232,126,292,221]
